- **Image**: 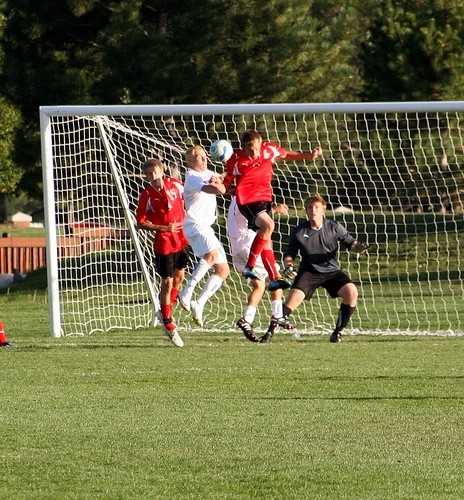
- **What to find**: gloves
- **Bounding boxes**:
[348,241,369,254]
[283,265,297,279]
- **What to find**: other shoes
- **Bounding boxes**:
[0,342,13,348]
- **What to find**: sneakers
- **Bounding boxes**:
[268,278,292,291]
[162,324,184,347]
[155,310,163,324]
[329,333,342,343]
[270,315,295,330]
[236,318,259,342]
[190,300,204,326]
[178,290,190,311]
[260,332,272,343]
[242,268,262,281]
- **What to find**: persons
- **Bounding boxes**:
[255,197,370,343]
[226,195,296,343]
[136,159,190,347]
[209,129,323,292]
[177,146,230,325]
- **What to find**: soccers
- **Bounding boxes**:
[209,139,233,162]
